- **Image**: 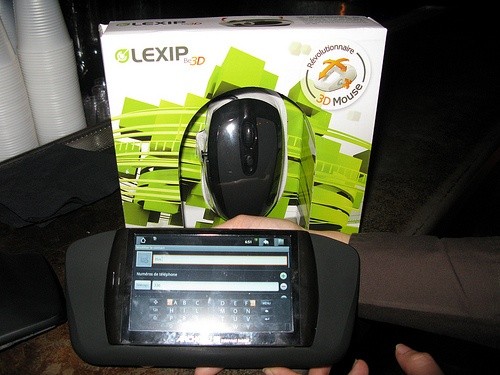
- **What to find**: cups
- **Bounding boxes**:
[0,0,39,163]
[83,77,111,128]
[13,0,87,148]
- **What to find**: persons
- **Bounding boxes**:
[192,214,498,375]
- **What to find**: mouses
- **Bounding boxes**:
[203,99,287,221]
